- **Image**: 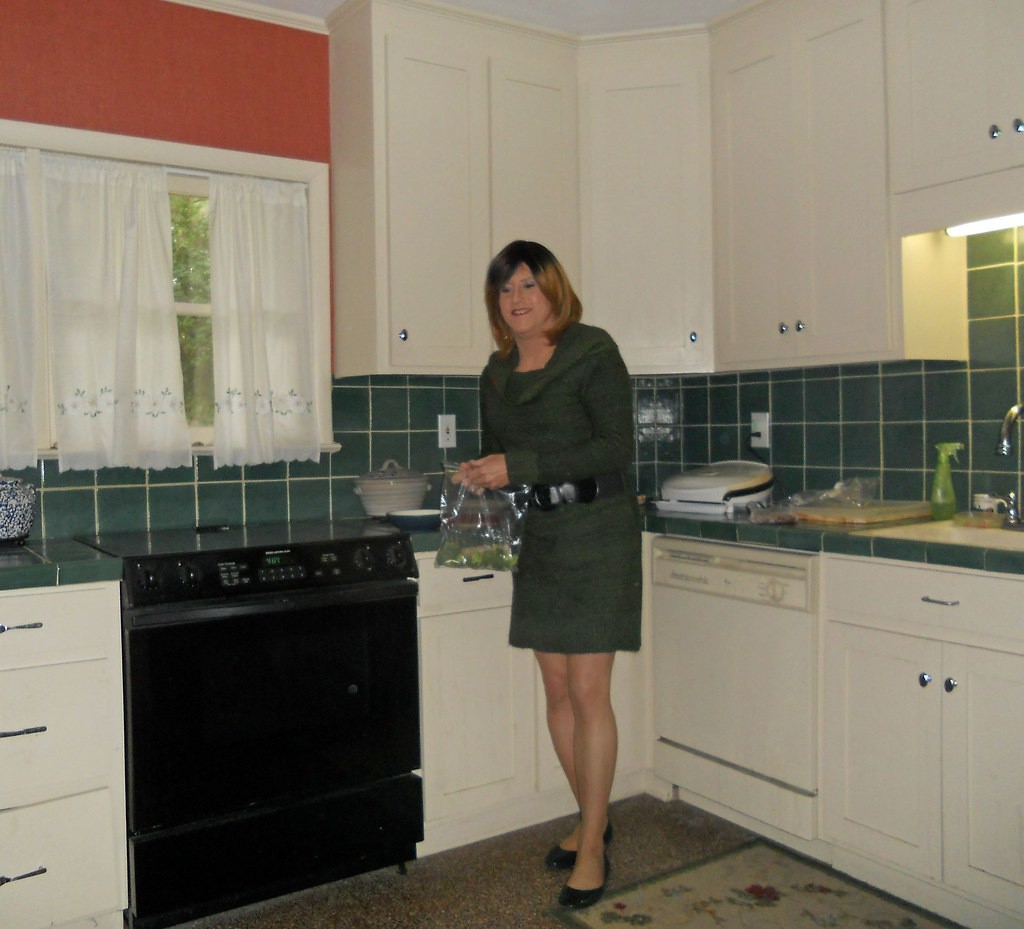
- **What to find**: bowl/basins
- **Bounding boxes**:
[352,460,434,516]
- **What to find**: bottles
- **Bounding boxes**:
[0,477,36,540]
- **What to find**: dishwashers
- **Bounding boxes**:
[650,535,820,841]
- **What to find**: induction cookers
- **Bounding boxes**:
[74,518,419,612]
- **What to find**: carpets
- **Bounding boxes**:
[544,838,970,929]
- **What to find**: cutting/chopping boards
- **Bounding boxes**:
[794,497,932,525]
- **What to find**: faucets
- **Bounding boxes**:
[995,403,1024,531]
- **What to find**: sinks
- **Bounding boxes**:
[849,519,1024,551]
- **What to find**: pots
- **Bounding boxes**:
[660,459,775,515]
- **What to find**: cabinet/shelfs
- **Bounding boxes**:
[413,549,648,861]
[819,550,1024,929]
[0,579,129,929]
[320,0,1024,375]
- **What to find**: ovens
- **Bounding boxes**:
[120,581,425,928]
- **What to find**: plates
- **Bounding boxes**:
[386,508,447,517]
[953,510,1005,528]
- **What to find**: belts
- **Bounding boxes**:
[513,470,622,512]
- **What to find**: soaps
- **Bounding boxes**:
[954,511,1005,528]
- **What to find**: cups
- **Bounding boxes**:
[973,493,1008,514]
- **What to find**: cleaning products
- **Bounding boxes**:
[931,443,964,520]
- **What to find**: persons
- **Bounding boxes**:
[450,240,644,910]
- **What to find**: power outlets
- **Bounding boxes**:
[750,411,770,448]
[438,415,457,448]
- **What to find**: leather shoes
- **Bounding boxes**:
[547,817,611,870]
[560,854,609,908]
